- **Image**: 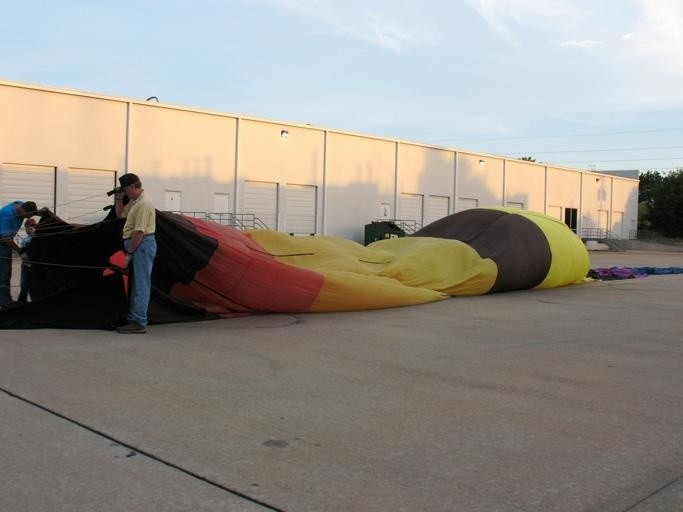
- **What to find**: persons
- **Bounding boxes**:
[1,201,38,303]
[113,174,157,333]
[15,219,37,301]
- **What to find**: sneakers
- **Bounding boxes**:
[117,322,146,333]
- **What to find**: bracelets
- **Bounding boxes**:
[126,248,133,254]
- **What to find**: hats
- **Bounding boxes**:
[119,174,139,187]
